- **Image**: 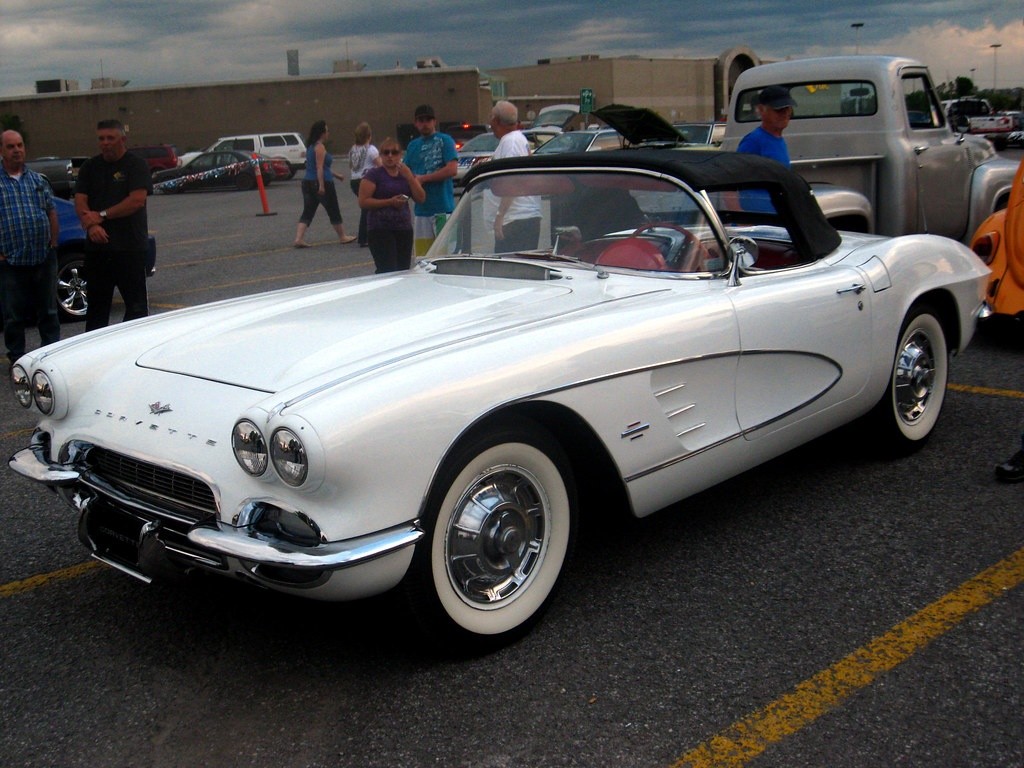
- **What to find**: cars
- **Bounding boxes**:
[968,155,1024,318]
[532,128,617,156]
[998,111,1024,146]
[54,196,156,323]
[8,147,993,658]
[446,124,492,152]
[589,103,726,150]
[452,105,581,181]
[128,144,178,173]
[151,150,275,194]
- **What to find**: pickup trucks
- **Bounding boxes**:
[176,132,309,181]
[24,157,91,200]
[940,97,1013,152]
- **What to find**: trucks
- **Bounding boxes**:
[630,55,1022,247]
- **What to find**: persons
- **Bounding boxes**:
[401,104,458,257]
[0,130,61,364]
[294,120,357,248]
[724,86,798,226]
[75,118,149,332]
[483,101,543,254]
[348,123,383,248]
[358,136,426,274]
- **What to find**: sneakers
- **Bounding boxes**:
[995,450,1024,482]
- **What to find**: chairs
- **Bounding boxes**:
[595,237,666,271]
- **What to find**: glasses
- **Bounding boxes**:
[383,150,399,155]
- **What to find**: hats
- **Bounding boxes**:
[758,86,797,109]
[415,105,434,118]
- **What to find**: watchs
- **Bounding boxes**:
[496,211,504,217]
[100,210,107,219]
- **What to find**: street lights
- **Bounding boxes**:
[990,44,1002,88]
[851,23,863,56]
